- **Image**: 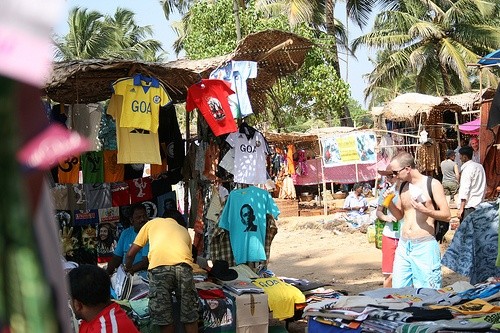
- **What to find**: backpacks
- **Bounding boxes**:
[400,176,449,241]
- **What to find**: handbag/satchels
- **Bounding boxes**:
[375,188,389,249]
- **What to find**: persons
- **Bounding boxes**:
[104,202,150,282]
[124,208,202,333]
[469,137,480,164]
[97,222,119,256]
[323,134,376,164]
[201,297,234,328]
[382,151,451,289]
[66,264,140,333]
[343,182,370,233]
[457,147,487,225]
[376,163,405,286]
[440,149,461,195]
[453,137,466,172]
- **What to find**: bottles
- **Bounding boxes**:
[393,220,399,231]
[382,181,397,208]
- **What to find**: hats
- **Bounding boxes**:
[378,164,393,176]
[446,150,456,156]
[207,259,238,281]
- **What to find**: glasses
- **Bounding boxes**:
[392,166,411,176]
[386,174,394,179]
